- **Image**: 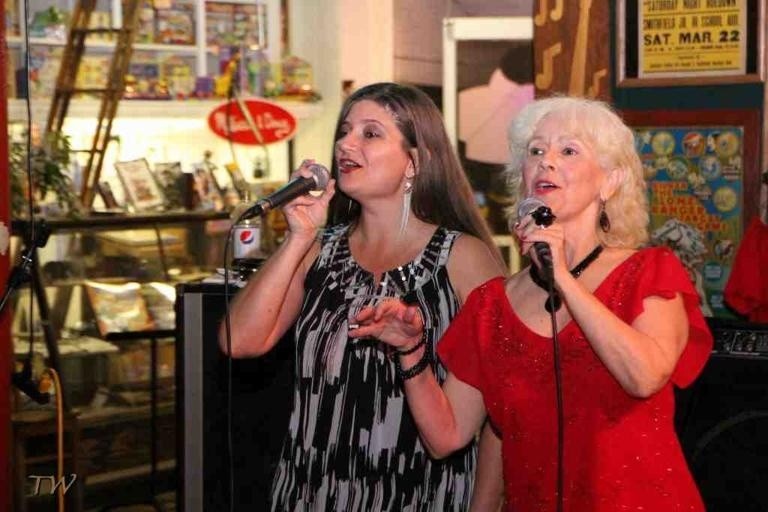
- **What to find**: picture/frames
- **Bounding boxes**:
[616,110,763,321]
[438,14,535,274]
[615,0,764,89]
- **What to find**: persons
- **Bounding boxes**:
[348,96,712,511]
[219,83,512,512]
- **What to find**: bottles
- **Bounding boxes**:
[231,188,263,271]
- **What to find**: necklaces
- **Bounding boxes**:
[528,244,603,312]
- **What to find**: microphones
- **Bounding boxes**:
[513,196,559,291]
[233,163,331,224]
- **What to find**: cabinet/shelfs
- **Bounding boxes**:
[12,209,263,512]
[7,0,322,120]
